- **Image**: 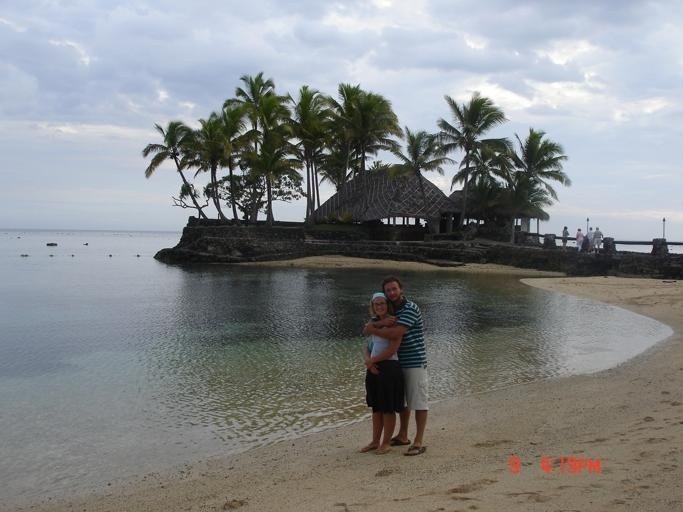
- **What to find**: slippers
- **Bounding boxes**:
[391,436,411,446]
[405,444,426,456]
[375,445,393,455]
[359,442,380,453]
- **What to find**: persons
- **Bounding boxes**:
[562,226,568,251]
[358,292,404,455]
[575,228,584,253]
[586,227,594,253]
[593,226,603,253]
[363,275,429,456]
